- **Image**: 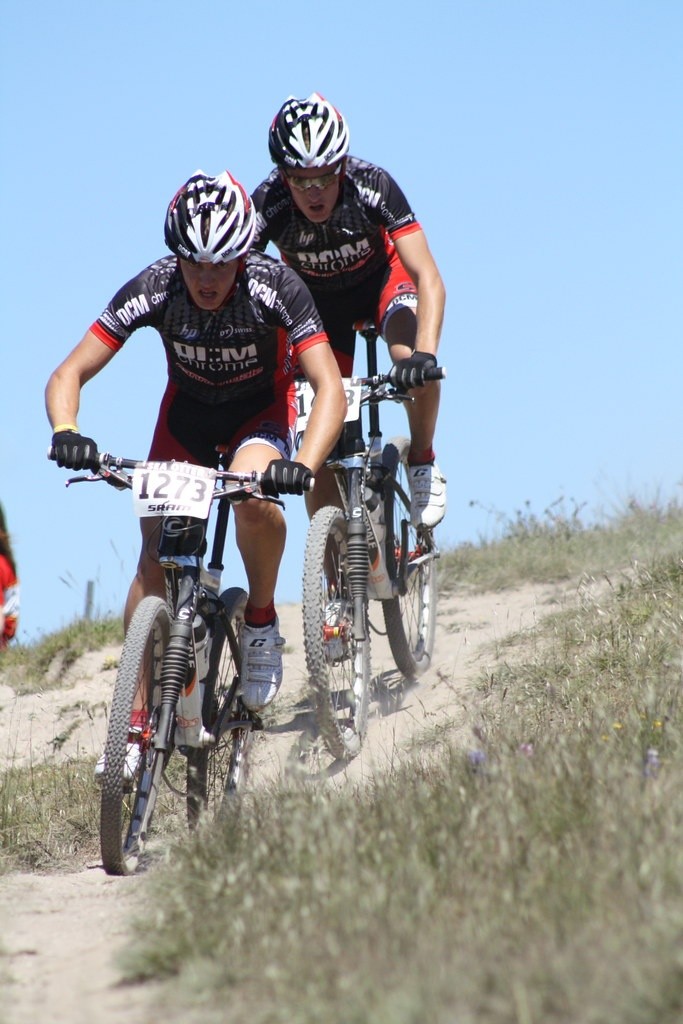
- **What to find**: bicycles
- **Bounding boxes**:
[48,444,317,875]
[294,320,446,763]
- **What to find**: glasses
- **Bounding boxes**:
[283,160,343,191]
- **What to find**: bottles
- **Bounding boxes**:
[190,616,211,683]
[363,486,386,544]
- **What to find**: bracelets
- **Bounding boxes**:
[56,425,78,432]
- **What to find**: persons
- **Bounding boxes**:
[44,169,346,791]
[247,94,446,659]
[0,528,21,650]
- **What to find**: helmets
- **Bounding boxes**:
[164,171,256,263]
[269,92,350,168]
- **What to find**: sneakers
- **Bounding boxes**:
[323,598,348,661]
[408,461,446,529]
[241,614,286,711]
[93,727,144,791]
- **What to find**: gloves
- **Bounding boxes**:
[51,426,100,476]
[388,351,438,404]
[261,459,314,498]
